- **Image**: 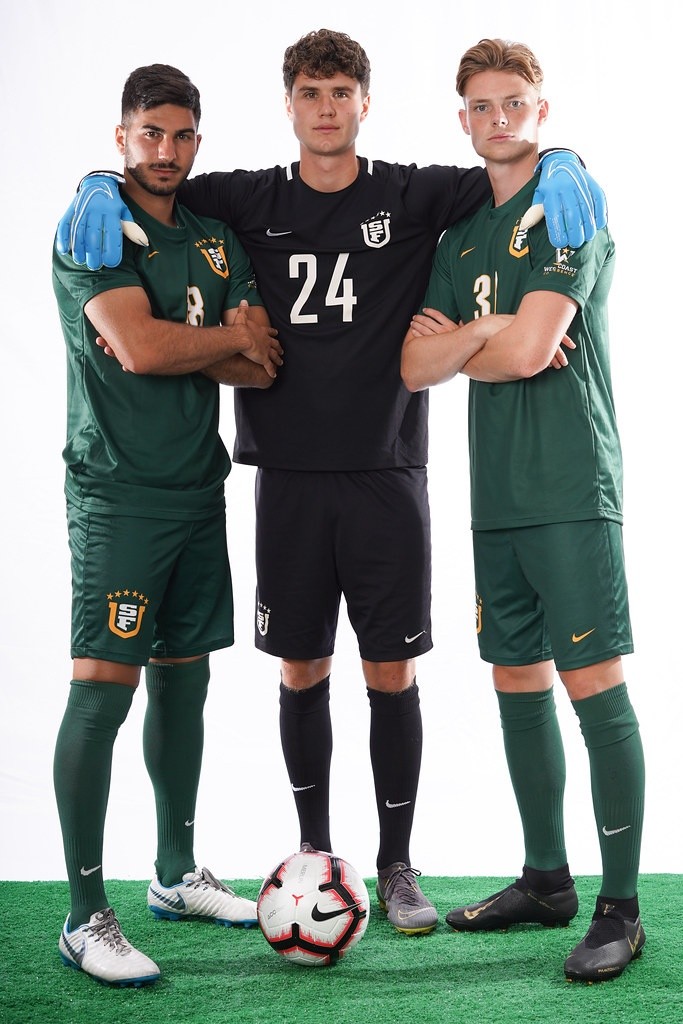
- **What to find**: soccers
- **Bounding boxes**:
[257,848,370,967]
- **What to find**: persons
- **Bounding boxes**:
[50,64,259,984]
[56,30,609,936]
[400,37,647,980]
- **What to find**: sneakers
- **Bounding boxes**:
[563,904,646,982]
[448,879,578,931]
[60,907,160,987]
[148,861,259,928]
[375,862,438,937]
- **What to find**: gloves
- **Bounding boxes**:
[517,149,608,248]
[55,174,148,270]
